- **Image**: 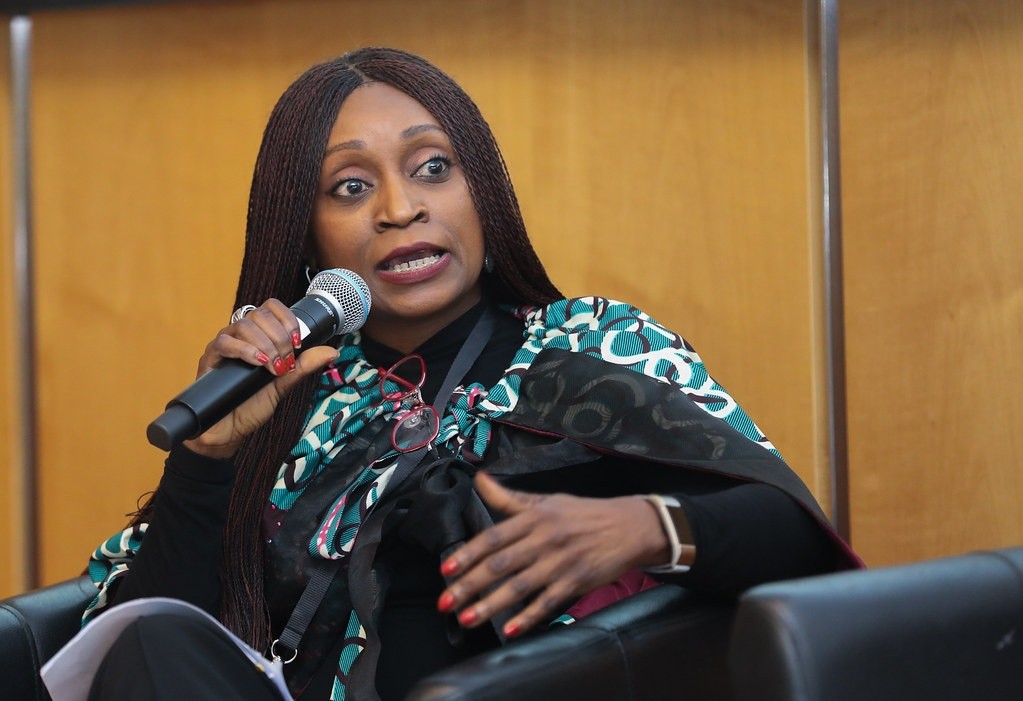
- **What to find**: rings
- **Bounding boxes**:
[231,305,255,324]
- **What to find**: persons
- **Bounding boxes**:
[83,48,866,700]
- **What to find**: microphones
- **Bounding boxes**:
[146,270,372,452]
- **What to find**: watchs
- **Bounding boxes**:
[630,494,695,574]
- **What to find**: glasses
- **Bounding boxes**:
[377,354,439,456]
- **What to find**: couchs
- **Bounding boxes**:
[0,583,694,701]
[729,546,1023,701]
